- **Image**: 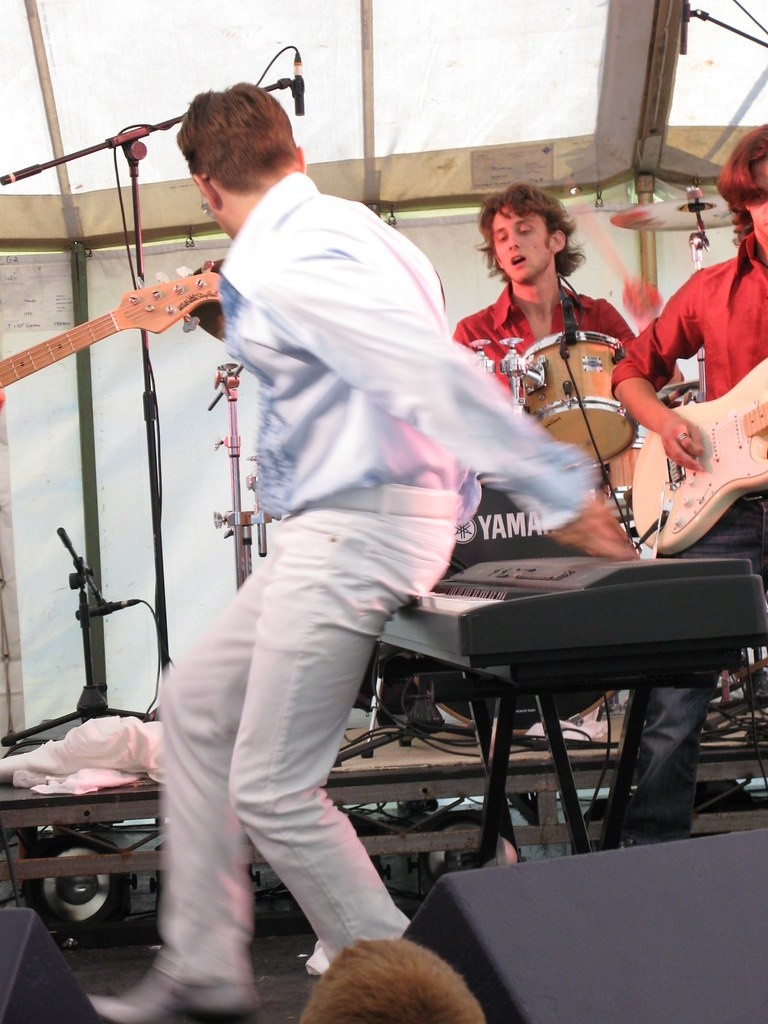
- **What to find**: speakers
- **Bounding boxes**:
[400,826,768,1024]
[0,835,130,1024]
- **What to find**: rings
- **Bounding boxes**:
[677,433,688,441]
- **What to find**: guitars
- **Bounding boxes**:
[0,266,230,391]
[629,345,768,558]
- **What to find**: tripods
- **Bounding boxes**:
[1,530,151,748]
[329,670,549,766]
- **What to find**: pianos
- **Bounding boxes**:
[374,550,768,696]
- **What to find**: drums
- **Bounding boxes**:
[518,327,642,468]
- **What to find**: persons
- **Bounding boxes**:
[298,939,486,1024]
[449,183,689,417]
[598,123,768,850]
[84,84,640,1023]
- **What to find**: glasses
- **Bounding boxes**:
[199,173,214,222]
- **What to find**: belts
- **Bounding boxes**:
[306,484,462,521]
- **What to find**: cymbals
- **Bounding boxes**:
[608,191,742,235]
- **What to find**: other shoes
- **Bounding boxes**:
[88,965,261,1023]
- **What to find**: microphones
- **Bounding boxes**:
[294,54,304,116]
[557,280,577,346]
[75,599,140,620]
